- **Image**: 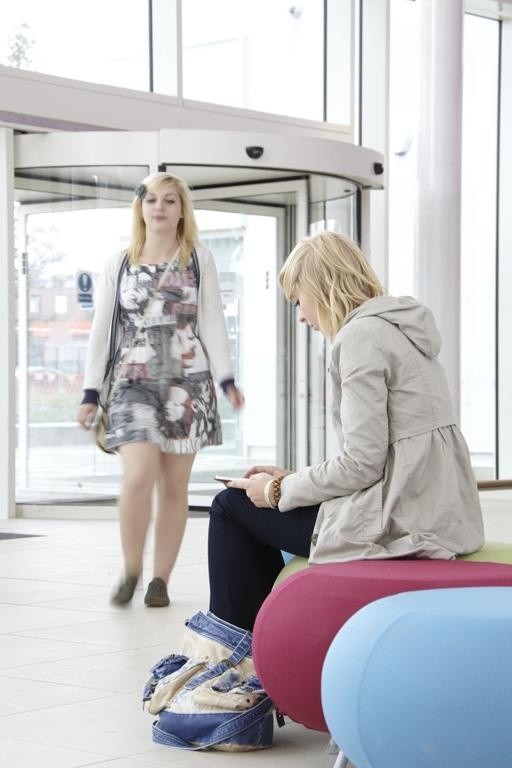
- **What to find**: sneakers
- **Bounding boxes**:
[111,569,138,604]
[144,578,170,608]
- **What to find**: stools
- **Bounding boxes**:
[321,585,510,763]
[271,541,510,588]
[252,560,507,732]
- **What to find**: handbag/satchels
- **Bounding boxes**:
[89,247,182,455]
[143,609,286,753]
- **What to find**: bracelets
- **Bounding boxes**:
[264,479,276,510]
[273,476,283,506]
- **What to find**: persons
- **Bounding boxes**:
[208,232,485,634]
[76,171,245,608]
